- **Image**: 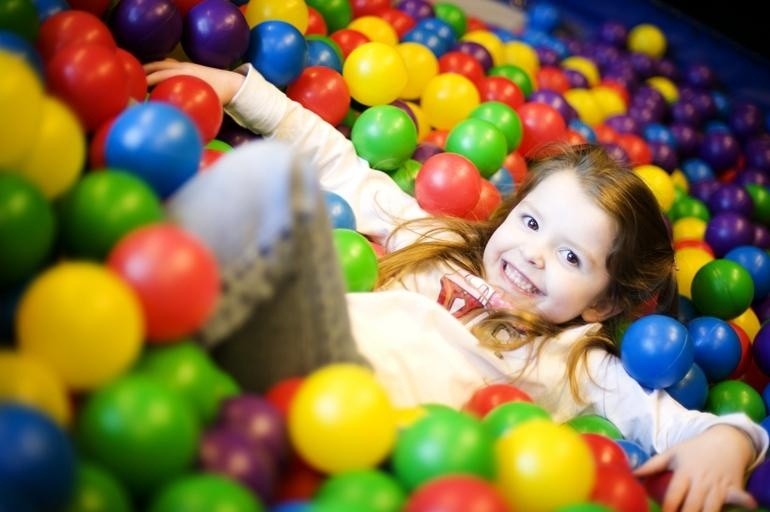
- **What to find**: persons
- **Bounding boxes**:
[142,57,770,512]
